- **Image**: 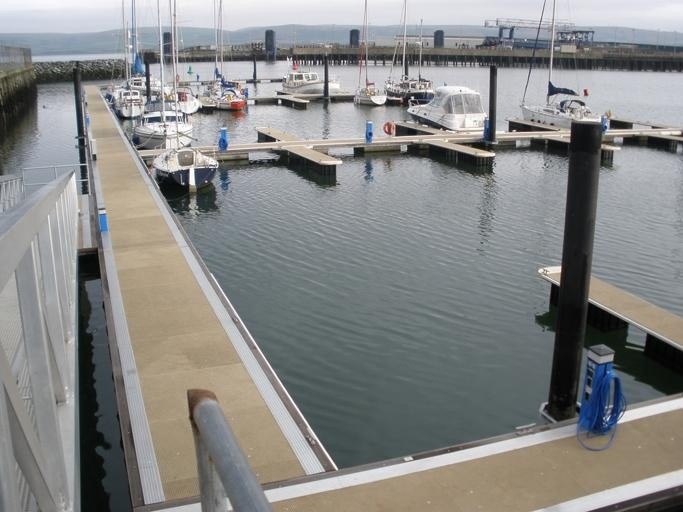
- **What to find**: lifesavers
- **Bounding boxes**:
[384,122,395,135]
[176,74,179,82]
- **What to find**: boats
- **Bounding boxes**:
[406,83,487,133]
[275,69,340,97]
[353,0,435,105]
[520,0,608,133]
[108,0,249,190]
